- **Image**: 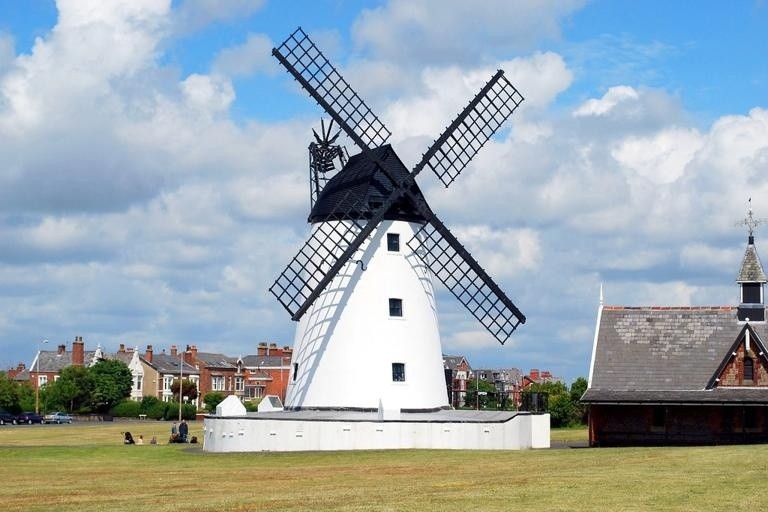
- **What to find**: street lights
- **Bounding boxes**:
[178,348,192,422]
[280,356,291,404]
[476,370,485,410]
[515,373,524,410]
[36,339,49,415]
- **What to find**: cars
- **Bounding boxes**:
[0,410,17,425]
[44,411,73,424]
[16,412,44,425]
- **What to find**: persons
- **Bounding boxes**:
[168,419,199,443]
[150,435,157,443]
[137,434,145,444]
